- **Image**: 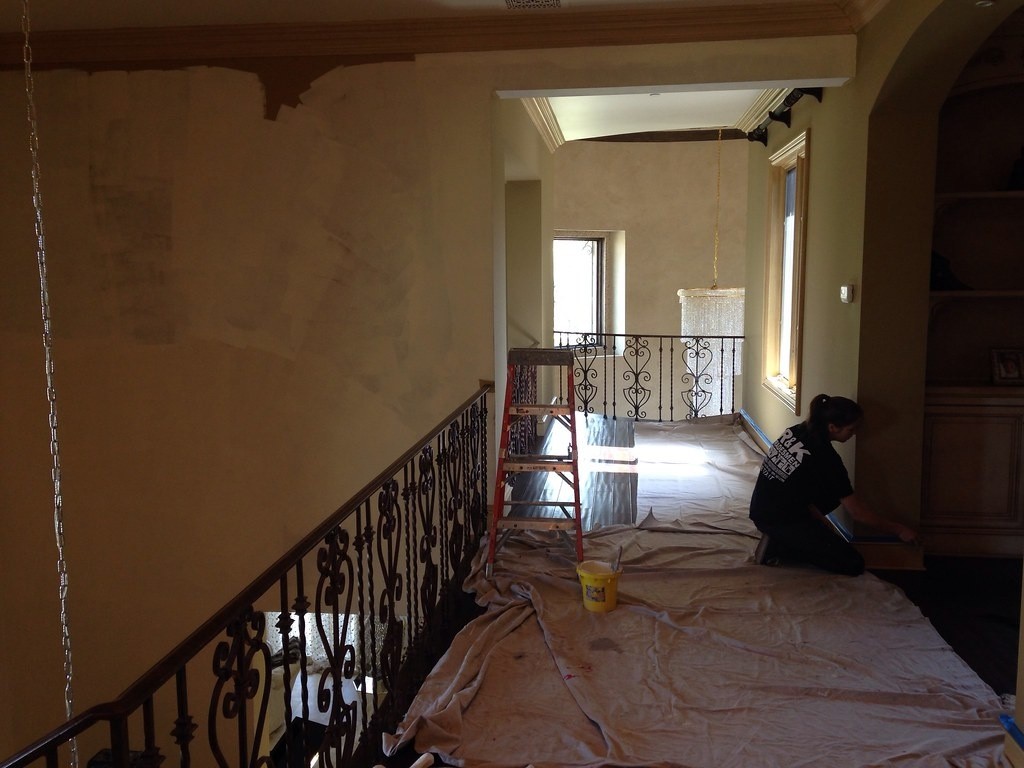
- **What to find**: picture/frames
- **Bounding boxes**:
[990,347,1024,387]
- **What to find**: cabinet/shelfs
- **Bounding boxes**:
[928,189,1024,298]
[921,389,1024,559]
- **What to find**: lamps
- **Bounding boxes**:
[678,128,745,419]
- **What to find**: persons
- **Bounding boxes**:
[749,394,924,578]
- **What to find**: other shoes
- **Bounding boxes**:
[755,533,774,565]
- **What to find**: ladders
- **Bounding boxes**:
[484,349,585,577]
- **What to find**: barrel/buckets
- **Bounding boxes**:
[576,559,623,612]
